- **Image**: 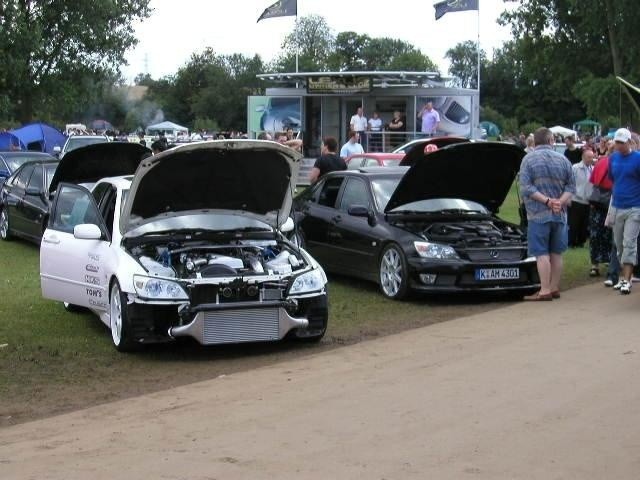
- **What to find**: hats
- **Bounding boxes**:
[614,129,632,143]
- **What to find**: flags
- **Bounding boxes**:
[433,0,479,21]
[257,0,297,23]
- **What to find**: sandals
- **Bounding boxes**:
[589,268,599,276]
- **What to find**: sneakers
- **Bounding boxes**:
[604,275,640,293]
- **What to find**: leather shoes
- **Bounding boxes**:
[524,290,560,301]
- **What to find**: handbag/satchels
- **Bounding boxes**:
[589,186,611,208]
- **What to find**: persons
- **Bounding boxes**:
[340,131,365,157]
[501,127,640,301]
[309,137,347,184]
[389,109,404,146]
[418,102,440,136]
[350,107,367,134]
[67,127,303,150]
[367,111,382,152]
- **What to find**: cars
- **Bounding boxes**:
[340,135,475,170]
[293,142,544,301]
[548,143,586,158]
[37,137,330,355]
[0,134,114,247]
